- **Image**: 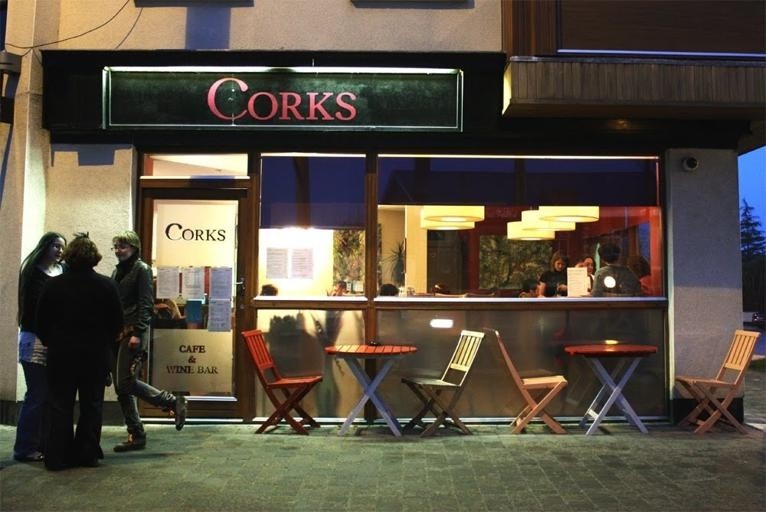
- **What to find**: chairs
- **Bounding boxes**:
[676,329,762,434]
[239,329,323,435]
[401,329,487,437]
[494,331,570,433]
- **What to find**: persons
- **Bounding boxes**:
[260,282,277,298]
[108,225,188,452]
[13,233,66,462]
[520,243,651,299]
[327,282,349,298]
[379,284,399,298]
[36,230,124,471]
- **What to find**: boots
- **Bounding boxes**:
[113,432,147,453]
[162,396,186,431]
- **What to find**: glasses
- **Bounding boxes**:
[111,245,130,251]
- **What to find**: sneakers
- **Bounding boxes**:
[13,449,105,471]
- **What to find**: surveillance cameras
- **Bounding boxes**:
[683,157,699,171]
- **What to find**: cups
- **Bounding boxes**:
[398,287,408,297]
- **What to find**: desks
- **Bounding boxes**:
[565,344,659,434]
[324,344,419,436]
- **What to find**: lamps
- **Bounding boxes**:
[522,210,577,232]
[507,221,556,241]
[419,219,476,231]
[421,205,485,221]
[539,206,600,224]
